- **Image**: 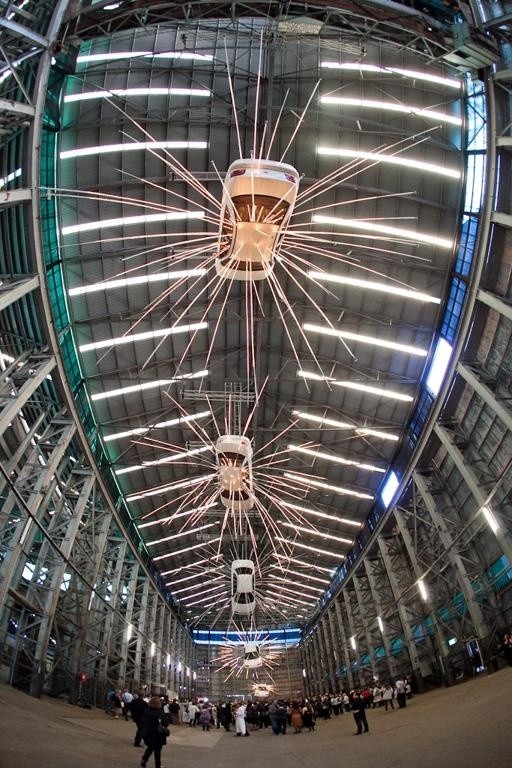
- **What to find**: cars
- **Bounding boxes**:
[243,643,263,669]
[214,156,301,283]
[214,434,254,511]
[254,684,270,697]
[231,559,256,616]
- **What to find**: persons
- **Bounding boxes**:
[108,691,216,748]
[217,700,316,737]
[308,679,406,735]
[141,696,171,768]
[411,632,512,695]
